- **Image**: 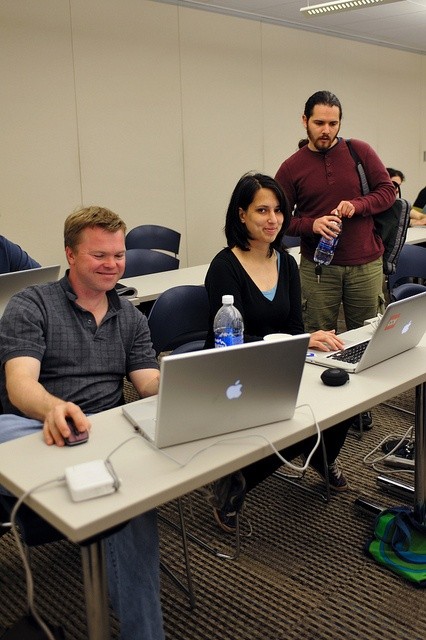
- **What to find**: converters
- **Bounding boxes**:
[63,460,117,502]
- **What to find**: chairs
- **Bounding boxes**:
[386,243,426,300]
[147,285,209,357]
[124,225,181,278]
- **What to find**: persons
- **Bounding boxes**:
[409,187,426,227]
[203,172,349,534]
[386,167,403,197]
[275,89,398,433]
[0,206,166,640]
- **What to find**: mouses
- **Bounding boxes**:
[60,415,89,445]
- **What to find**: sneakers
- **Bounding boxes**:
[308,458,348,492]
[355,412,374,430]
[213,494,237,532]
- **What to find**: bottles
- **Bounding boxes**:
[314,210,342,266]
[213,294,244,348]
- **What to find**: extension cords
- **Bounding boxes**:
[385,452,415,468]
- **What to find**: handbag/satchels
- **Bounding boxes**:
[374,180,411,275]
[364,507,426,585]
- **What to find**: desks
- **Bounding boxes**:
[0,298,426,640]
[103,221,425,305]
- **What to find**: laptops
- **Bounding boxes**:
[122,333,311,450]
[306,291,426,375]
